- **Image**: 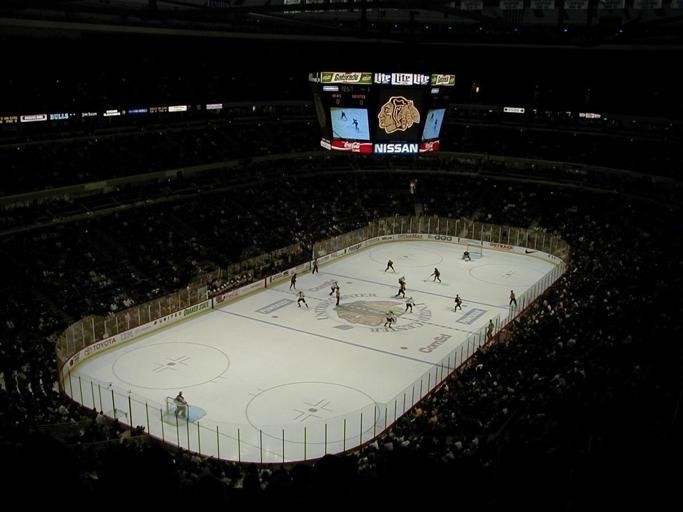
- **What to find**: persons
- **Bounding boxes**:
[2,105,682,512]
